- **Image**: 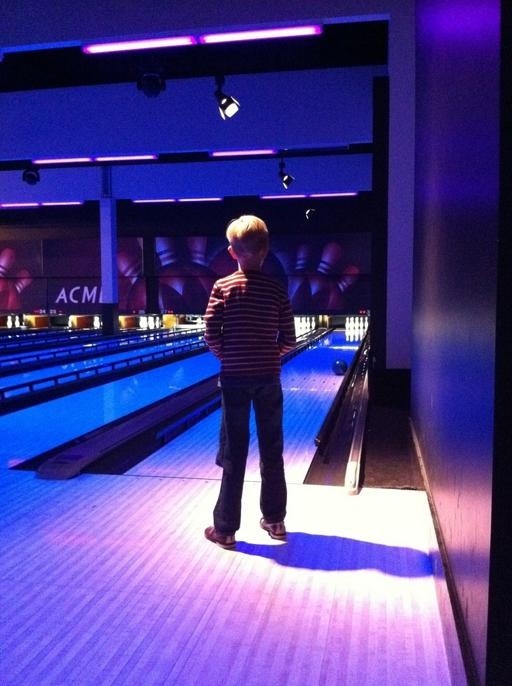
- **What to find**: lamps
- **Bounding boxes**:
[214,87,242,120]
[280,170,296,190]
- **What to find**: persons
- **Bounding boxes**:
[201,215,296,549]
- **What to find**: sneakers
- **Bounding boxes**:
[261,517,286,539]
[205,527,237,549]
